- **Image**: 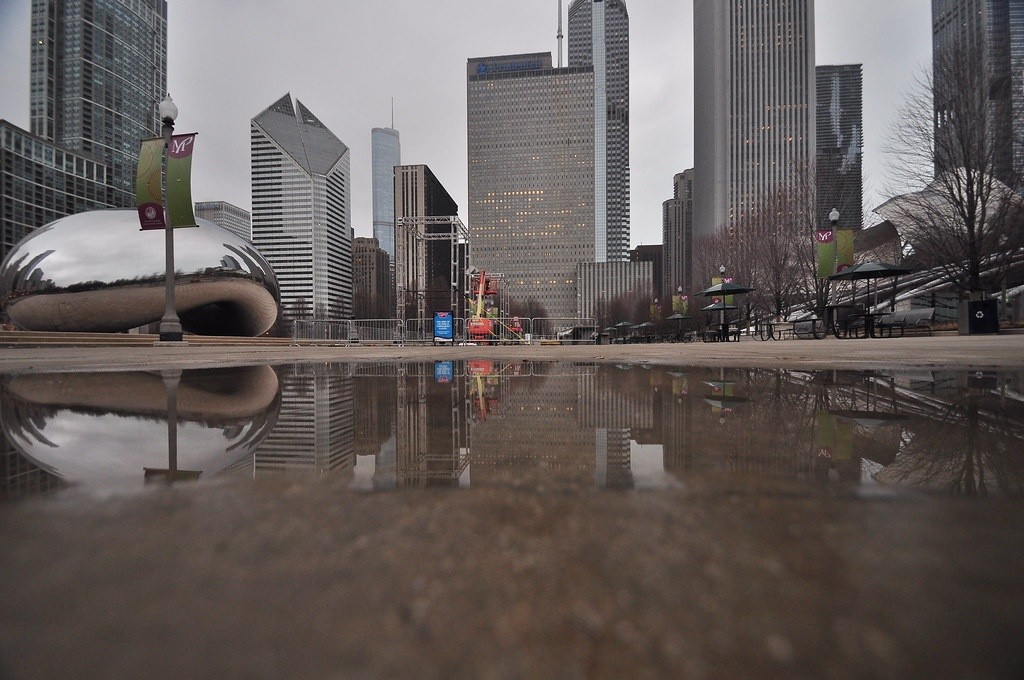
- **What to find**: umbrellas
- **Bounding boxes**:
[639,322,656,329]
[828,261,912,314]
[666,313,693,333]
[614,321,632,336]
[700,302,738,318]
[604,327,618,338]
[694,282,756,320]
[629,325,646,334]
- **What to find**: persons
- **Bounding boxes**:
[629,332,639,343]
[679,330,691,342]
[648,332,654,342]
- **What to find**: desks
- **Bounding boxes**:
[711,323,735,341]
[811,305,841,340]
[762,324,779,341]
[848,313,891,338]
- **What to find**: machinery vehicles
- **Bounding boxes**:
[468,270,494,345]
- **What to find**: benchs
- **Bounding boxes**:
[751,330,789,341]
[879,307,935,337]
[789,319,892,339]
[697,331,741,342]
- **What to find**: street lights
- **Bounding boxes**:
[654,298,659,337]
[829,208,840,331]
[719,265,726,341]
[678,368,683,404]
[827,369,840,481]
[719,368,726,425]
[159,94,184,341]
[678,286,682,335]
